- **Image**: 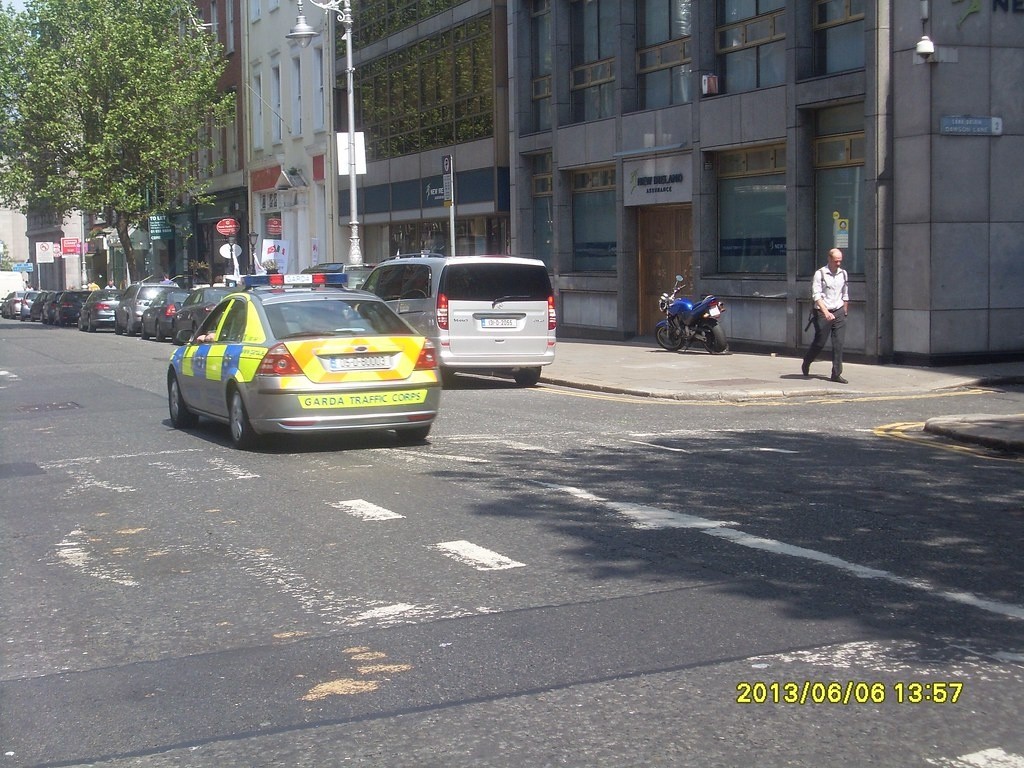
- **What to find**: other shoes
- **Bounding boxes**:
[802,359,809,376]
[831,375,848,384]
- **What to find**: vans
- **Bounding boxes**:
[358,253,556,386]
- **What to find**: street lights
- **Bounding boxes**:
[284,0,364,289]
[247,230,259,275]
[226,231,236,275]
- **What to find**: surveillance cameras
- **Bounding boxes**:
[916,40,934,59]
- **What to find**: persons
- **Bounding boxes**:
[87,280,100,291]
[196,332,216,344]
[104,279,117,289]
[159,272,174,285]
[802,248,849,385]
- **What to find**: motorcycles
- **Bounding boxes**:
[655,275,726,354]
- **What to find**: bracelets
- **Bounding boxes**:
[204,334,208,342]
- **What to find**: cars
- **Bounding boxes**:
[0,282,244,346]
[167,273,445,450]
